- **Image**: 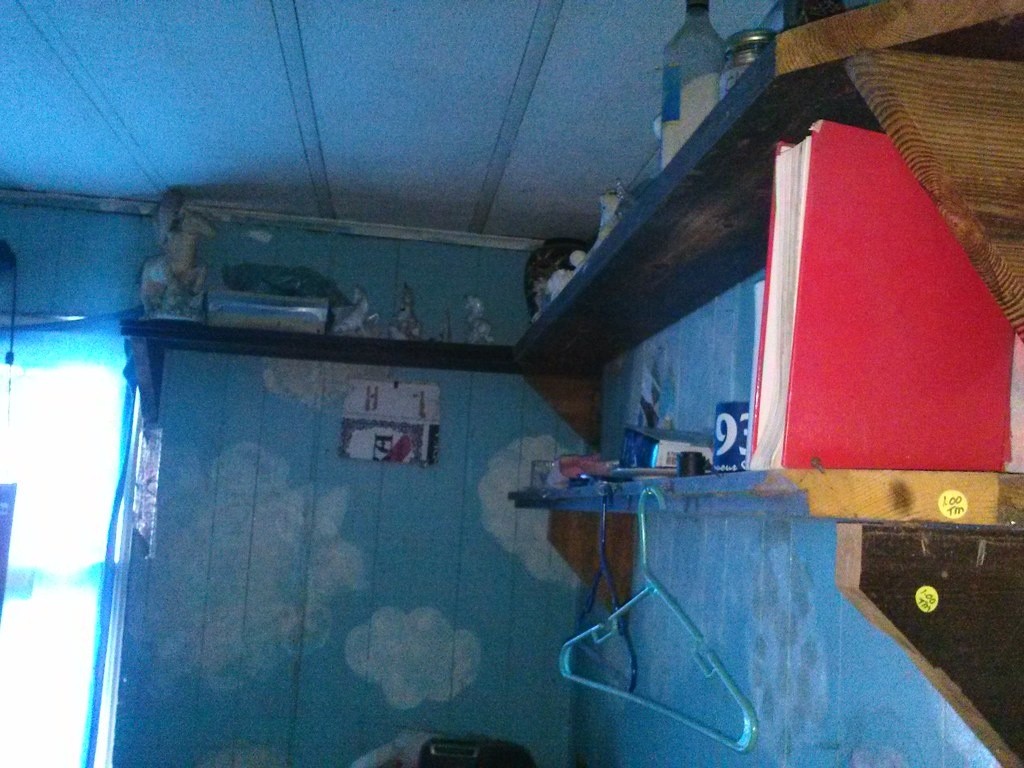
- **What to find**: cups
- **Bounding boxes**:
[712,402,749,473]
[677,452,705,477]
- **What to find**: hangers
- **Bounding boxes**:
[566,486,638,693]
[559,486,760,753]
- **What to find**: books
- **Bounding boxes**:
[747,119,1014,469]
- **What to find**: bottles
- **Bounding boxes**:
[660,0,725,171]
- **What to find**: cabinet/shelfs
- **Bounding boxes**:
[506,0,1024,768]
